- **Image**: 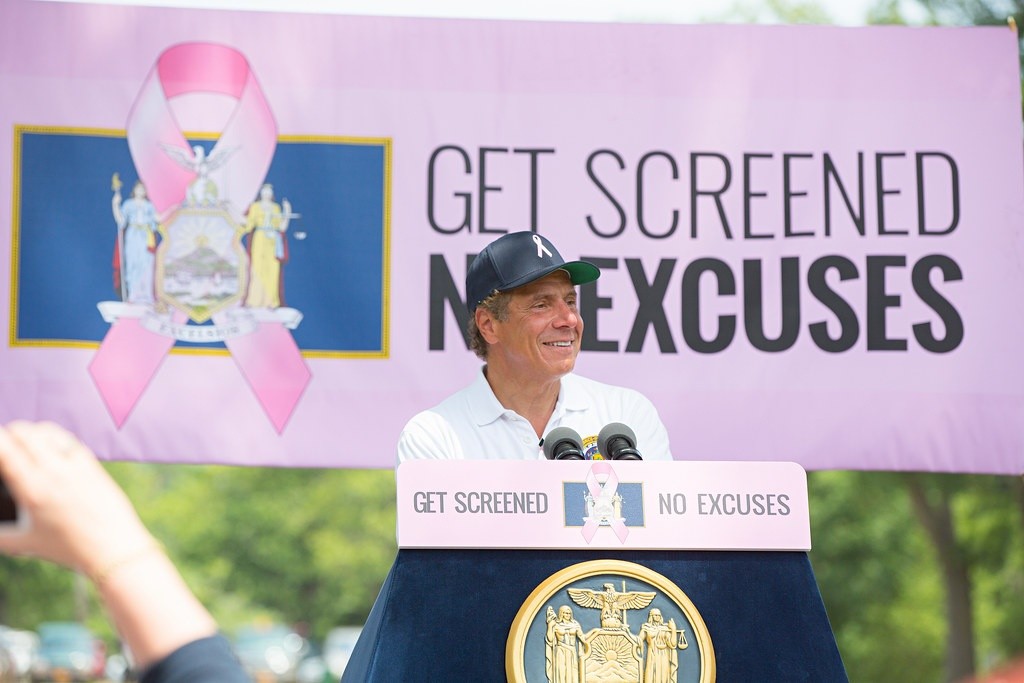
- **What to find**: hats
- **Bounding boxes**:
[465,231,601,317]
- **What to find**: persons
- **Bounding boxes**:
[0,418,257,683]
[396,231,673,465]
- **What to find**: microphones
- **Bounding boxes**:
[597,422,643,461]
[543,426,585,460]
